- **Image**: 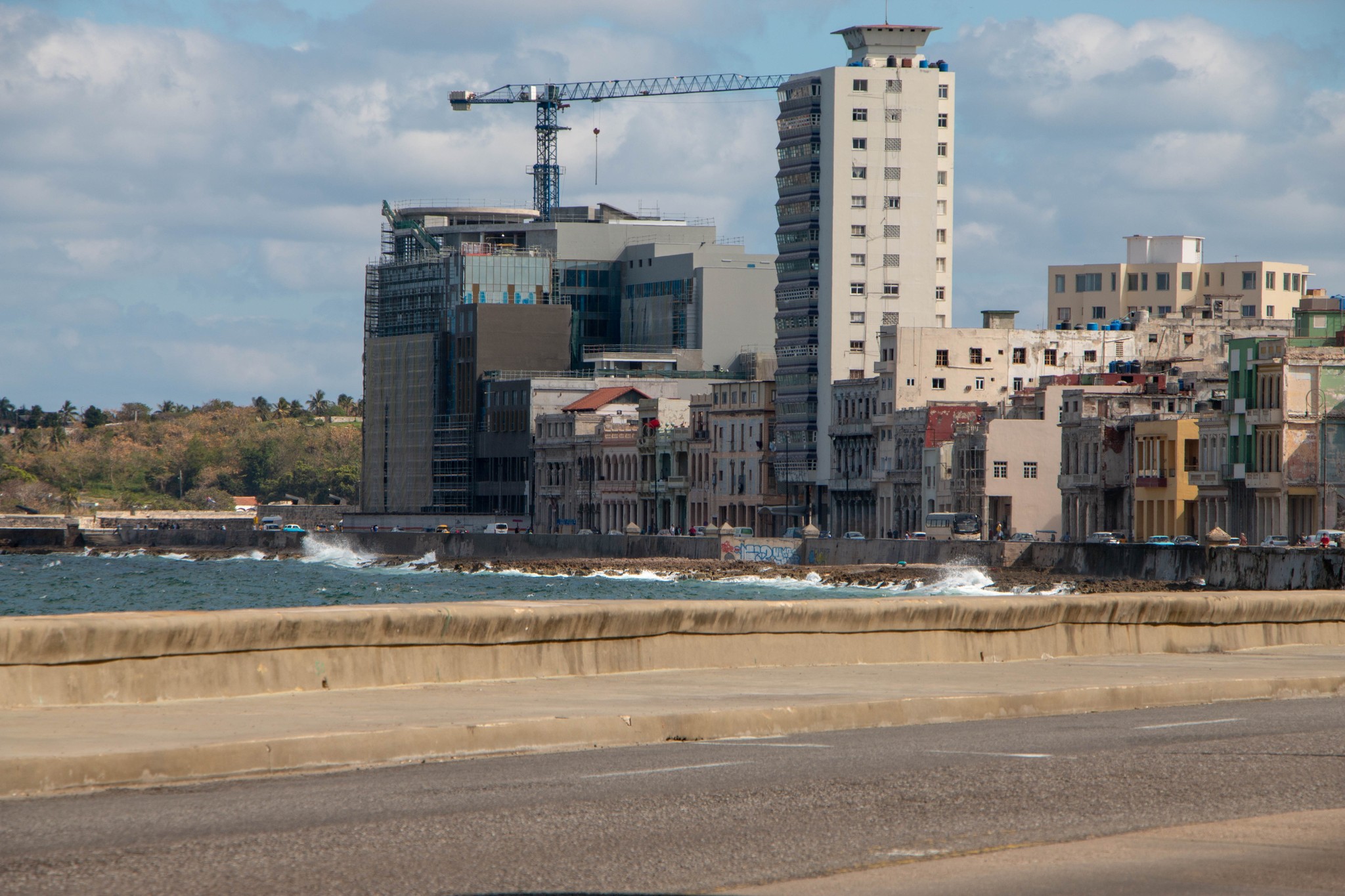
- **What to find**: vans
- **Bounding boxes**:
[783,527,803,538]
[1307,529,1345,548]
[734,527,754,538]
[693,526,706,536]
[483,523,508,533]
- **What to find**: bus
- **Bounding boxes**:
[925,512,983,541]
[258,516,283,526]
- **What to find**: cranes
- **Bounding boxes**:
[450,73,795,223]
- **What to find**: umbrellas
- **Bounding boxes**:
[513,516,523,526]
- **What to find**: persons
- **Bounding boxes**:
[442,528,448,533]
[881,526,912,540]
[670,523,697,536]
[604,366,619,377]
[641,523,658,535]
[1296,533,1311,545]
[594,526,608,535]
[1034,532,1055,542]
[549,523,559,534]
[315,523,343,532]
[112,522,121,535]
[371,524,378,532]
[1238,533,1247,546]
[252,522,285,531]
[515,526,533,534]
[1315,533,1330,548]
[136,522,180,529]
[718,367,724,379]
[534,248,539,257]
[989,522,1003,541]
[1260,540,1265,546]
[208,523,226,531]
[1060,532,1072,542]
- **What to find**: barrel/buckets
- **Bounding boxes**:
[1171,366,1182,376]
[1166,382,1180,394]
[1110,360,1141,374]
[1177,379,1184,391]
[1055,318,1132,331]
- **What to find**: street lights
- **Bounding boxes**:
[1304,388,1326,528]
[521,419,847,534]
[952,410,971,512]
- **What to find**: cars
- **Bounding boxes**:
[819,531,832,539]
[1008,532,1036,541]
[608,531,622,535]
[264,524,281,531]
[1086,532,1127,544]
[283,524,305,532]
[421,526,469,533]
[1172,536,1200,546]
[1260,535,1291,547]
[911,532,926,540]
[1145,536,1174,545]
[389,527,404,532]
[576,529,602,535]
[843,531,866,539]
[314,524,327,532]
[657,530,675,536]
[1228,538,1241,546]
